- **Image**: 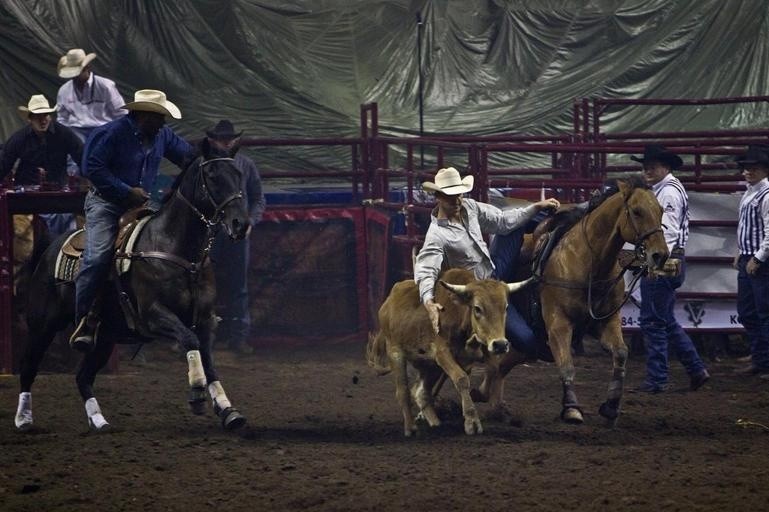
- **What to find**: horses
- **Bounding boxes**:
[469,172,670,423]
[14,135,252,432]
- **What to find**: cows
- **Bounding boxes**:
[365,267,534,437]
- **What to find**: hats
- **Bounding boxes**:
[119,89,182,120]
[422,167,474,196]
[206,119,244,138]
[57,48,96,78]
[734,150,768,164]
[631,143,682,170]
[19,94,59,122]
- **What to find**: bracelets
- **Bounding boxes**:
[752,254,763,267]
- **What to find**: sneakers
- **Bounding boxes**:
[639,381,669,392]
[683,368,709,390]
[215,339,253,354]
[733,364,758,375]
[74,334,93,351]
[539,345,554,362]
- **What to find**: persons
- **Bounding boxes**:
[0,94,86,299]
[69,87,198,348]
[180,115,267,356]
[417,162,563,405]
[637,142,710,396]
[54,46,131,189]
[733,151,768,379]
